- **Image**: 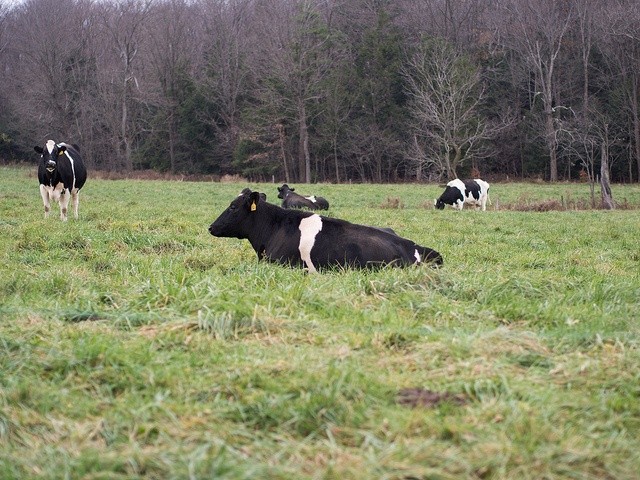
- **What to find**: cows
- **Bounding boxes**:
[34,139,87,222]
[433,178,491,212]
[275,184,329,211]
[207,187,443,274]
